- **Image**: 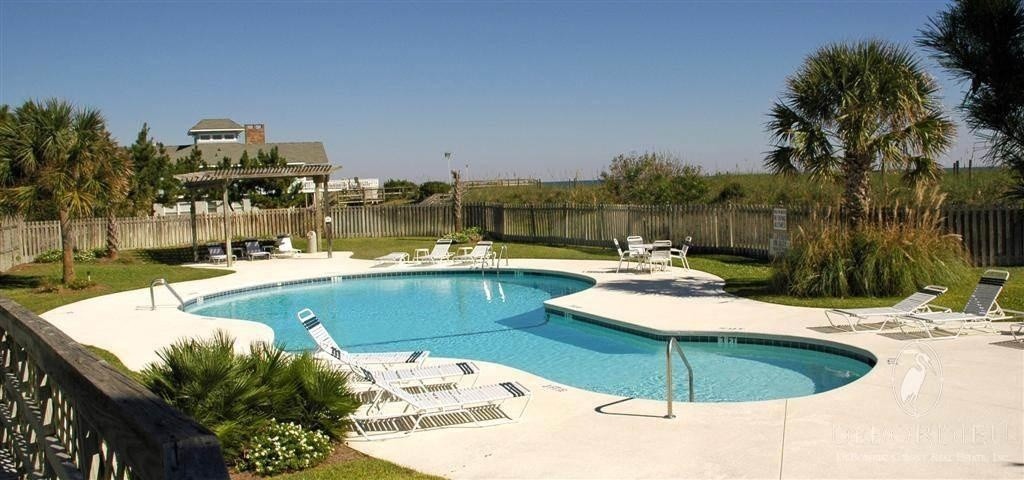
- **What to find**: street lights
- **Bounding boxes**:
[444,151,452,186]
[465,164,468,181]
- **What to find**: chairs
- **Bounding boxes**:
[611,231,693,277]
[818,266,1024,345]
[294,304,534,443]
[201,232,303,265]
[370,237,497,270]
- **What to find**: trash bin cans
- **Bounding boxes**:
[306,231,317,253]
[277,236,292,252]
